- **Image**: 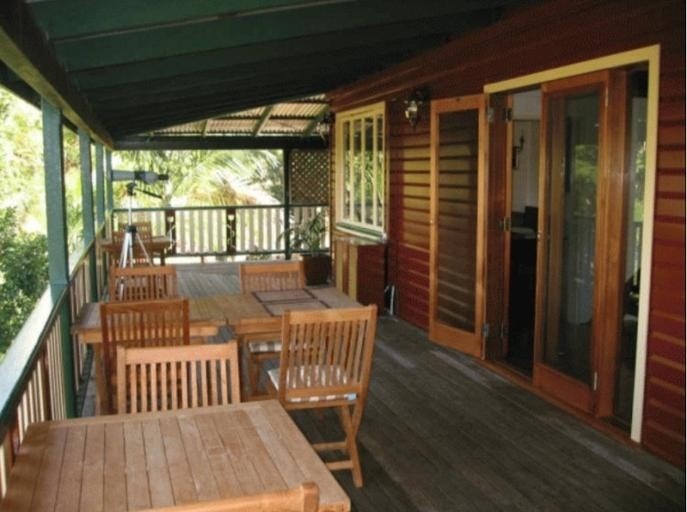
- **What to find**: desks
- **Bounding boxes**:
[71,298,226,412]
[102,235,172,296]
[208,287,366,403]
[15,397,351,512]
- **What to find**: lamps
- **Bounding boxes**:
[403,85,434,131]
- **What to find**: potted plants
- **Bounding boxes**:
[275,209,334,283]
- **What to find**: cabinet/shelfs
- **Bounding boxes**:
[336,234,380,301]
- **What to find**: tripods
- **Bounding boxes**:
[115,195,157,302]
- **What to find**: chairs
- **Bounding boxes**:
[239,260,317,397]
[114,342,241,410]
[267,304,379,489]
[100,299,191,409]
[108,265,180,295]
[112,222,155,266]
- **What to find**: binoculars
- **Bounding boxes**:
[108,171,169,185]
